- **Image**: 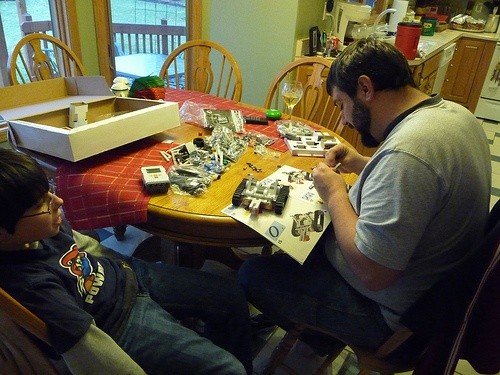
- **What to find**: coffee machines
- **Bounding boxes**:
[331,2,372,52]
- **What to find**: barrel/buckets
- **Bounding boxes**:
[394,22,422,60]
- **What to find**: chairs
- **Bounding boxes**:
[159,40,242,102]
[0,288,57,375]
[265,58,346,136]
[260,202,500,375]
[7,34,86,85]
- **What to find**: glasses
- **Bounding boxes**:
[16,184,55,219]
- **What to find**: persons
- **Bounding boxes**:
[0,145,251,375]
[235,37,492,370]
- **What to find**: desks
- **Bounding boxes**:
[35,90,361,313]
[114,53,184,79]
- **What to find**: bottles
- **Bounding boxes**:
[419,16,437,36]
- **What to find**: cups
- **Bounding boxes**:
[351,24,367,39]
[484,14,500,33]
[112,77,130,98]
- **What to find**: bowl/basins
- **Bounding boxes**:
[425,13,448,21]
[435,23,448,32]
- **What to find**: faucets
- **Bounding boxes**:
[367,9,396,40]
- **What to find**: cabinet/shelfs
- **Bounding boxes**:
[292,28,500,157]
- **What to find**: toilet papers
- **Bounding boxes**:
[388,0,409,32]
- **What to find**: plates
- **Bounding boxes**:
[453,26,485,32]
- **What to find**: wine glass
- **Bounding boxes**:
[281,81,304,125]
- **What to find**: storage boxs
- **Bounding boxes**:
[0,77,180,162]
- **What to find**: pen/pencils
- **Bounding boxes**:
[308,162,342,190]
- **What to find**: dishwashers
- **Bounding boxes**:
[432,43,456,96]
